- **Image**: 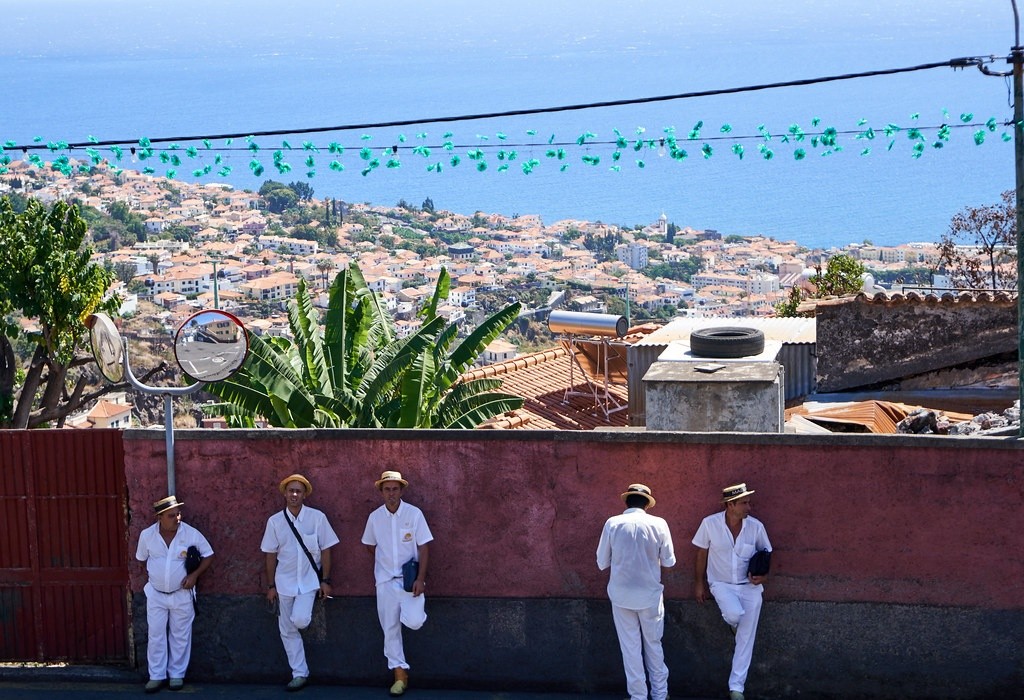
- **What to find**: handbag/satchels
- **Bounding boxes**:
[184,546,203,574]
[402,557,420,592]
[316,567,324,601]
[747,548,772,577]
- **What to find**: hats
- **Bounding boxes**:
[375,471,409,490]
[152,495,184,517]
[621,483,656,508]
[717,483,755,504]
[280,474,312,498]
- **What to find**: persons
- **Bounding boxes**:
[136,495,214,691]
[260,474,339,689]
[596,483,676,700]
[692,483,773,700]
[362,471,434,694]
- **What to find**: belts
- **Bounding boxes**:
[157,590,178,594]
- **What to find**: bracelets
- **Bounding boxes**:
[320,578,332,585]
[267,584,275,589]
[416,579,426,586]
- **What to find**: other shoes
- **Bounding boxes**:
[145,680,164,692]
[390,678,408,696]
[170,678,183,691]
[730,691,744,700]
[730,625,738,635]
[287,676,307,692]
